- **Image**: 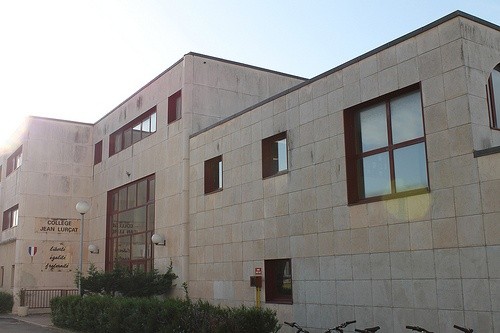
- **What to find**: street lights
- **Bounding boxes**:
[75,201,88,298]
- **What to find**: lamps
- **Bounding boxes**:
[88,244,100,254]
[151,234,166,247]
